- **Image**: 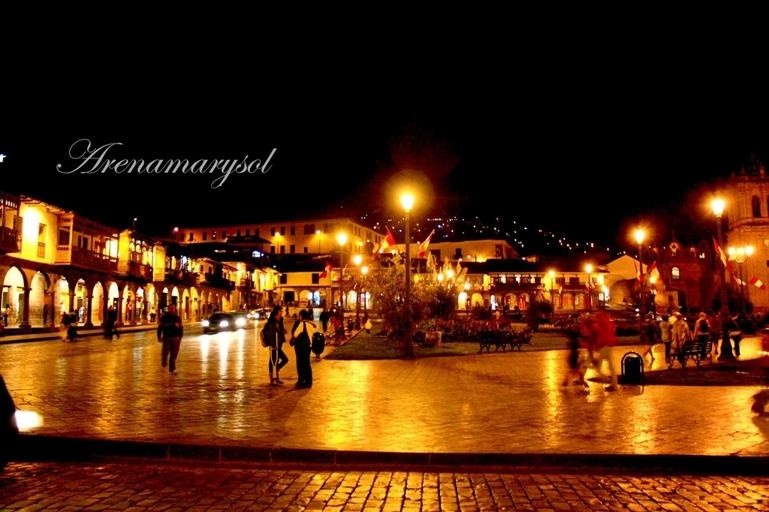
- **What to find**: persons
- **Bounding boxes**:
[103,303,119,340]
[258,300,346,390]
[66,307,81,341]
[3,299,11,328]
[42,303,51,325]
[156,302,184,377]
[149,306,156,323]
[473,291,768,391]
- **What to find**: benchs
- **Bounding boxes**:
[666,338,714,371]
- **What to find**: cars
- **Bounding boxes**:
[203,307,274,333]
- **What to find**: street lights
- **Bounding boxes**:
[397,189,417,317]
[337,230,347,316]
[584,263,594,309]
[438,270,471,317]
[711,198,740,362]
[132,217,137,229]
[629,224,647,340]
[726,241,755,311]
[353,254,362,330]
[359,266,369,327]
[548,268,557,318]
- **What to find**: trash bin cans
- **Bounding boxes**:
[620,352,645,385]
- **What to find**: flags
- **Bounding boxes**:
[416,230,435,259]
[318,260,332,281]
[366,233,396,259]
[634,238,769,292]
